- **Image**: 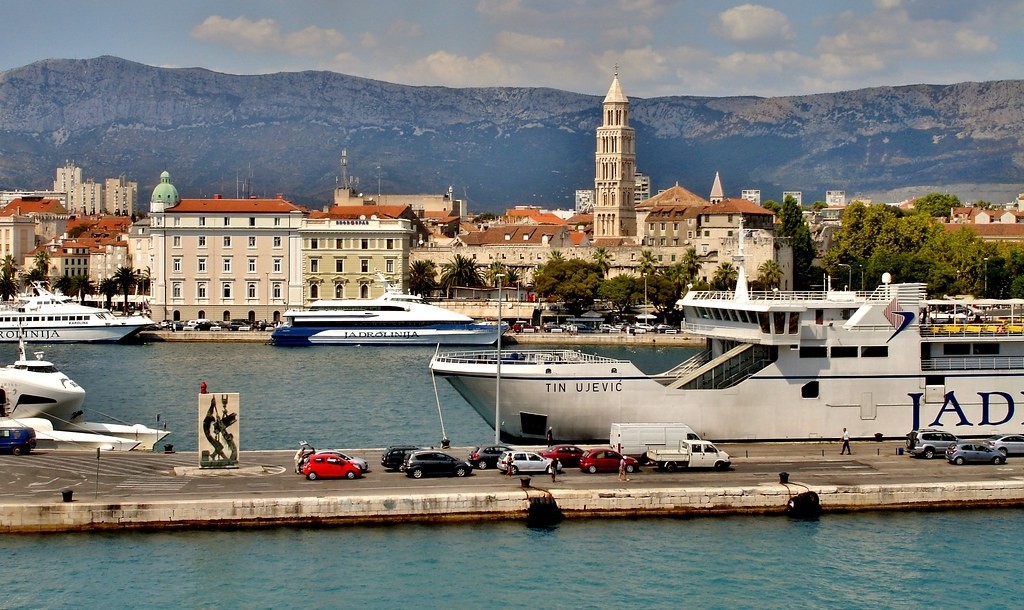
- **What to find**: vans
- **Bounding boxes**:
[0,426,37,456]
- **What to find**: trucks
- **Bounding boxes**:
[608,422,707,467]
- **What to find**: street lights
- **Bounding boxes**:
[838,264,852,292]
[859,264,864,297]
[983,258,989,298]
[643,272,648,325]
[516,279,523,321]
[494,273,507,445]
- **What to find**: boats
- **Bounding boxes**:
[0,322,173,452]
[0,280,157,346]
[426,211,1024,445]
[264,270,511,347]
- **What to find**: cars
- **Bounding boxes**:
[299,439,369,472]
[538,444,585,467]
[496,450,563,475]
[598,321,682,335]
[466,444,517,471]
[578,447,639,474]
[983,433,1024,457]
[945,442,1007,466]
[161,317,276,333]
[300,453,363,480]
[544,320,596,334]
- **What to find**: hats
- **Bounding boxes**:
[623,456,627,459]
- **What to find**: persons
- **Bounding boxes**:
[547,426,553,447]
[504,453,513,479]
[166,320,176,332]
[565,324,578,336]
[840,428,851,455]
[551,457,558,482]
[968,310,973,316]
[993,304,1000,309]
[618,456,630,482]
[293,447,305,475]
[975,316,983,323]
[251,319,267,331]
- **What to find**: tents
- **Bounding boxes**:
[72,294,151,308]
[581,310,603,317]
[635,313,657,319]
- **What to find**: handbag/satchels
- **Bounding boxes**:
[548,467,555,475]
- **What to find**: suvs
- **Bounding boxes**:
[381,444,445,473]
[905,428,960,460]
[513,320,538,333]
[399,450,475,479]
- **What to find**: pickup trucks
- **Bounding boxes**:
[646,439,732,473]
